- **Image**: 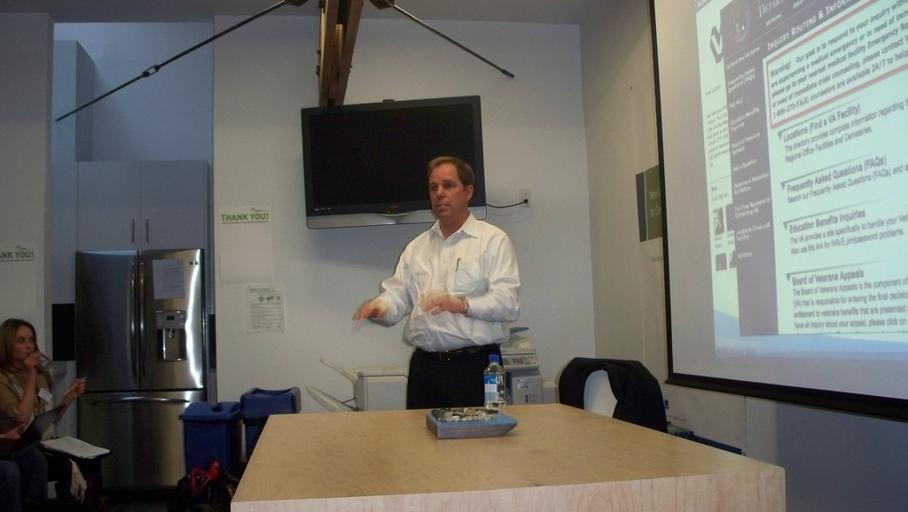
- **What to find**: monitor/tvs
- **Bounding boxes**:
[300,95,487,229]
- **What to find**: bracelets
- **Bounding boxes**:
[458,295,468,314]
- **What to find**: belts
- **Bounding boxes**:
[434,346,480,361]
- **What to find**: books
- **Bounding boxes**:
[39,435,111,460]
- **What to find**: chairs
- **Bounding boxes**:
[560,357,666,434]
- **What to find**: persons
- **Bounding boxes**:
[352,157,521,410]
[0,317,88,511]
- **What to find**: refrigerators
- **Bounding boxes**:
[72,248,205,491]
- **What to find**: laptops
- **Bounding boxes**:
[0,403,66,463]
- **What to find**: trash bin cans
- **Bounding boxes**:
[179,388,301,476]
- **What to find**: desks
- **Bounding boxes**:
[230,403,787,512]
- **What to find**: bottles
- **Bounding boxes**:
[27,350,55,375]
[483,354,507,413]
